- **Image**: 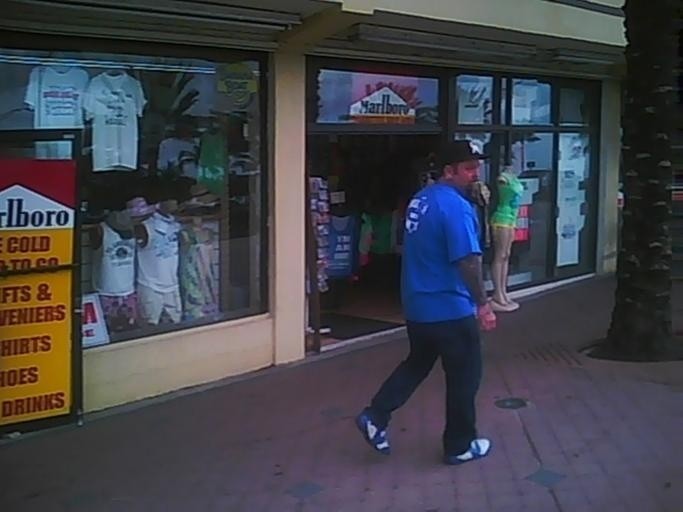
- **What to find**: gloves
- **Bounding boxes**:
[478,303,497,331]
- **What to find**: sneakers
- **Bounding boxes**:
[356,411,389,455]
[445,438,490,465]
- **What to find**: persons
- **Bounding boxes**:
[355,142,498,466]
[90,209,139,332]
[135,199,183,325]
[487,159,523,306]
[179,212,219,320]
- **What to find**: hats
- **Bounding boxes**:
[177,184,222,216]
[122,196,156,217]
[441,140,490,164]
